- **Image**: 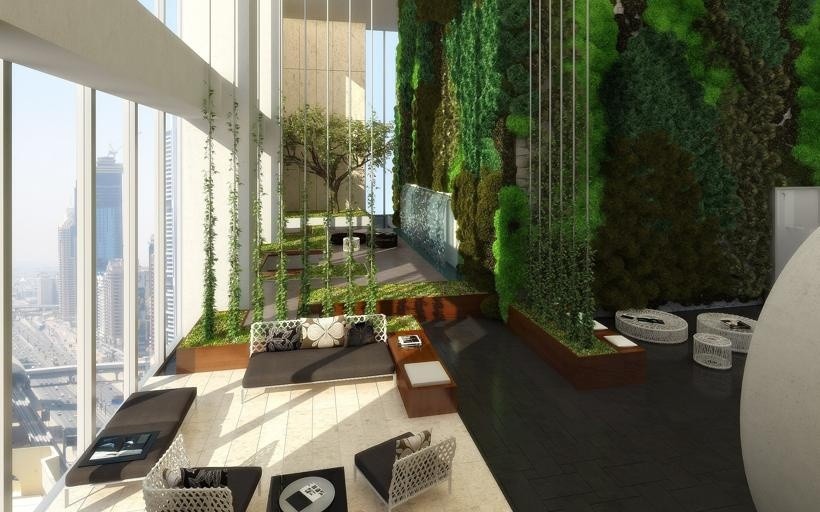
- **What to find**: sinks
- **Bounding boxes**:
[366,232,399,248]
[330,232,366,245]
[240,313,397,405]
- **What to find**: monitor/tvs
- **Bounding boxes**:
[77,431,160,467]
[720,320,751,329]
[285,482,326,512]
[398,335,422,347]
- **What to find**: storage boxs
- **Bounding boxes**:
[64,386,198,508]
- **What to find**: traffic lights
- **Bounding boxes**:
[179,466,231,489]
[344,321,375,349]
[162,468,183,488]
[299,317,345,349]
[264,328,300,353]
[393,430,431,476]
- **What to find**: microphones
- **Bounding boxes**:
[614,307,689,345]
[696,311,758,354]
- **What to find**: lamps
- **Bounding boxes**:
[352,429,457,512]
[141,432,262,512]
